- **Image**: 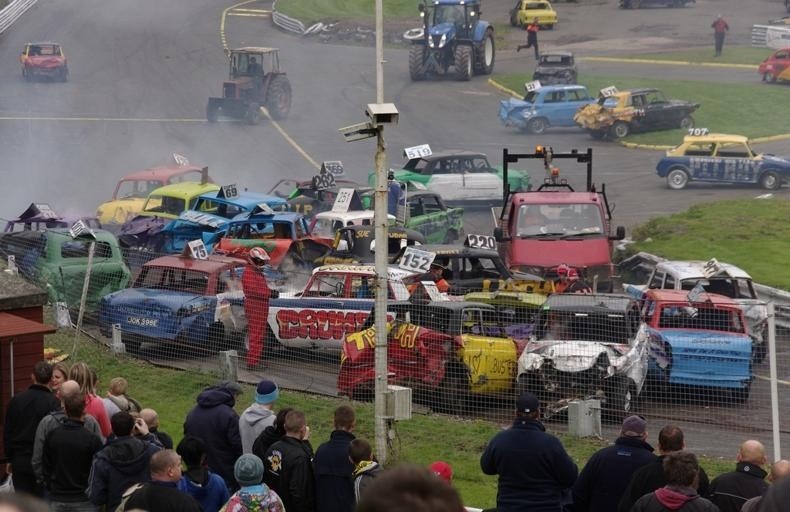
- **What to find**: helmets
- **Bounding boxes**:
[248,247,270,272]
[556,263,579,282]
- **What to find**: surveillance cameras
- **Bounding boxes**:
[366,102,399,126]
[338,121,379,142]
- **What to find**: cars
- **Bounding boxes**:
[515,291,652,428]
[645,258,770,363]
[95,160,626,361]
[335,298,521,416]
[626,287,758,404]
[656,127,789,190]
[511,1,557,31]
[533,53,577,88]
[20,43,68,82]
[0,200,134,324]
[575,86,700,138]
[758,47,790,84]
[500,79,595,135]
[389,144,506,211]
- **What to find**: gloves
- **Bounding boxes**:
[271,290,279,298]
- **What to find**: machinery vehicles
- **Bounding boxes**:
[405,1,494,79]
[206,44,293,126]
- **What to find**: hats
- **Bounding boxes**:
[622,415,647,437]
[222,381,243,394]
[234,454,264,485]
[517,393,539,413]
[431,263,444,270]
[256,380,277,402]
[431,462,452,482]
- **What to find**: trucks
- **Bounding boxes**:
[494,149,624,276]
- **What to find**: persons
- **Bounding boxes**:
[0,360,464,512]
[481,392,789,512]
[553,264,570,292]
[242,247,279,370]
[565,269,592,294]
[388,183,401,216]
[710,14,729,57]
[247,56,263,76]
[32,47,41,57]
[516,16,540,61]
[425,262,451,294]
[449,5,465,33]
